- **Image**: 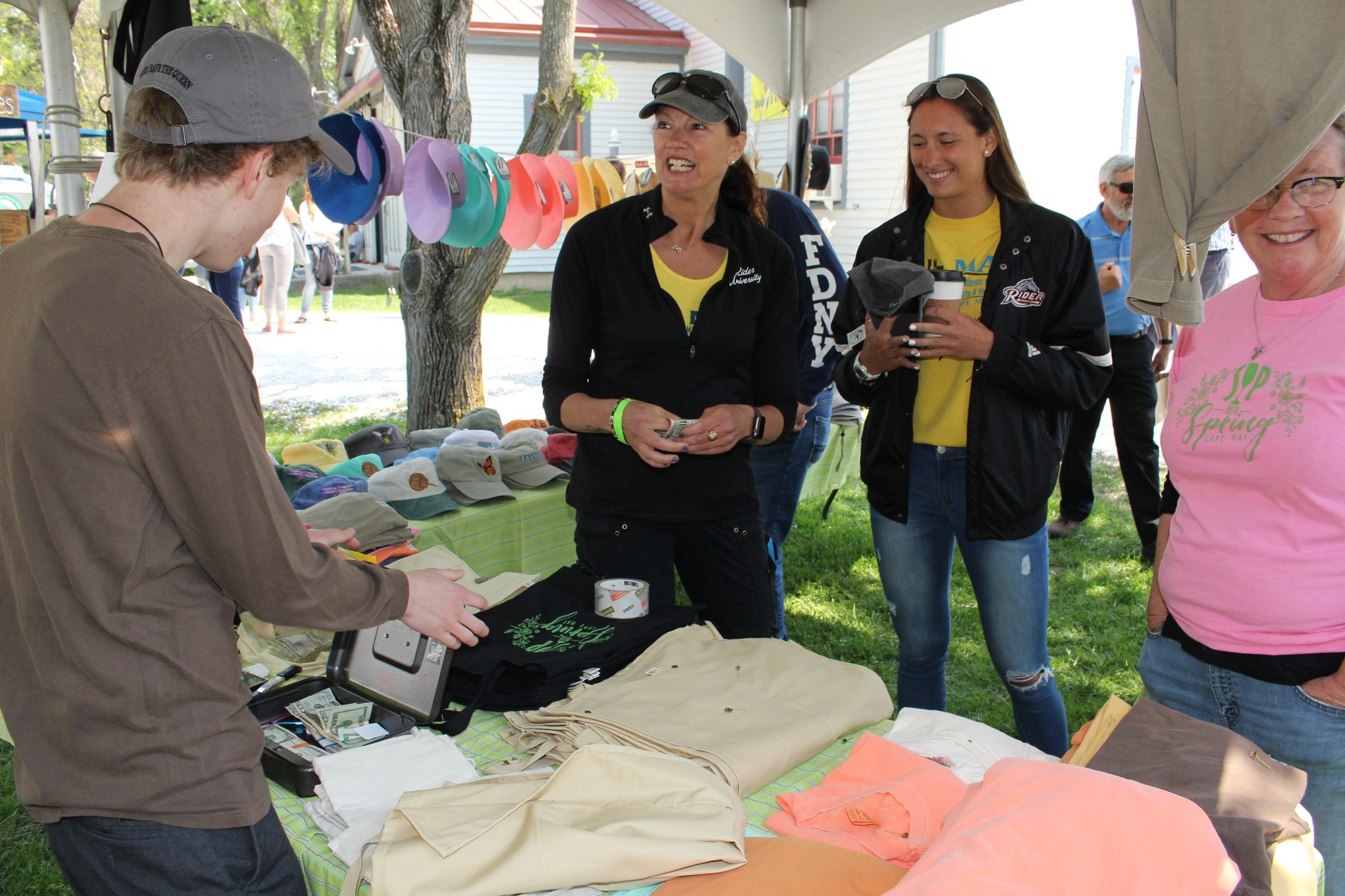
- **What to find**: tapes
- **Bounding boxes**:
[593,577,651,619]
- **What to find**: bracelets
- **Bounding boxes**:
[1158,339,1173,345]
[607,397,634,446]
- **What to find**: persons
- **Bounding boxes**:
[755,188,850,541]
[0,27,490,896]
[254,193,311,335]
[1049,155,1172,568]
[294,176,343,324]
[238,245,262,322]
[541,69,802,641]
[1138,112,1344,894]
[208,258,245,330]
[1200,219,1232,301]
[348,223,364,263]
[831,74,1115,758]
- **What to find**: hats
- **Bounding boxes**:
[795,117,830,202]
[847,256,935,362]
[121,22,356,176]
[304,112,626,252]
[638,69,748,132]
[266,407,578,554]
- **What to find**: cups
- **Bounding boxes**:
[921,268,966,338]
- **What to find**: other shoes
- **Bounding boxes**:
[263,326,272,333]
[1048,514,1081,539]
[249,321,261,327]
[324,317,336,322]
[277,328,295,334]
[294,317,307,324]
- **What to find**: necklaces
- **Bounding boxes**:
[89,202,164,258]
[1251,272,1340,360]
[668,233,702,253]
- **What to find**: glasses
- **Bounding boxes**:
[651,72,743,131]
[1246,176,1345,210]
[1110,181,1133,194]
[906,77,983,107]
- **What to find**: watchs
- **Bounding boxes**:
[738,405,766,443]
[853,350,888,380]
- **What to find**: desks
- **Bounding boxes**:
[799,424,863,520]
[269,703,894,895]
[408,481,578,584]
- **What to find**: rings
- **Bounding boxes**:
[865,316,872,322]
[708,429,717,441]
